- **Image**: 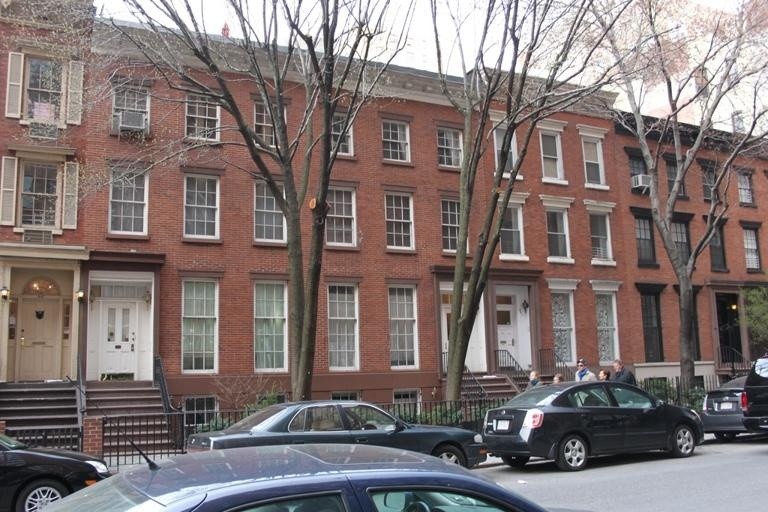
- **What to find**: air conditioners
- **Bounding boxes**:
[631,173,651,192]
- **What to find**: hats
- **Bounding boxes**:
[575,359,585,363]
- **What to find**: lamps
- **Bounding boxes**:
[76,287,85,304]
[0,286,10,301]
[522,299,529,312]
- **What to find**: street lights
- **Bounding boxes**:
[731,303,738,379]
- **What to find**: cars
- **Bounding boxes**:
[188,400,488,468]
[34,443,550,512]
[701,354,768,439]
[0,434,112,512]
[482,381,704,472]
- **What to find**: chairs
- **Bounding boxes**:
[583,395,601,406]
[311,418,337,430]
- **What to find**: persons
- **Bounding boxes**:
[575,356,597,405]
[553,373,564,381]
[524,369,544,392]
[599,368,610,382]
[610,357,637,388]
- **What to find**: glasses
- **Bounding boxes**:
[576,365,585,368]
